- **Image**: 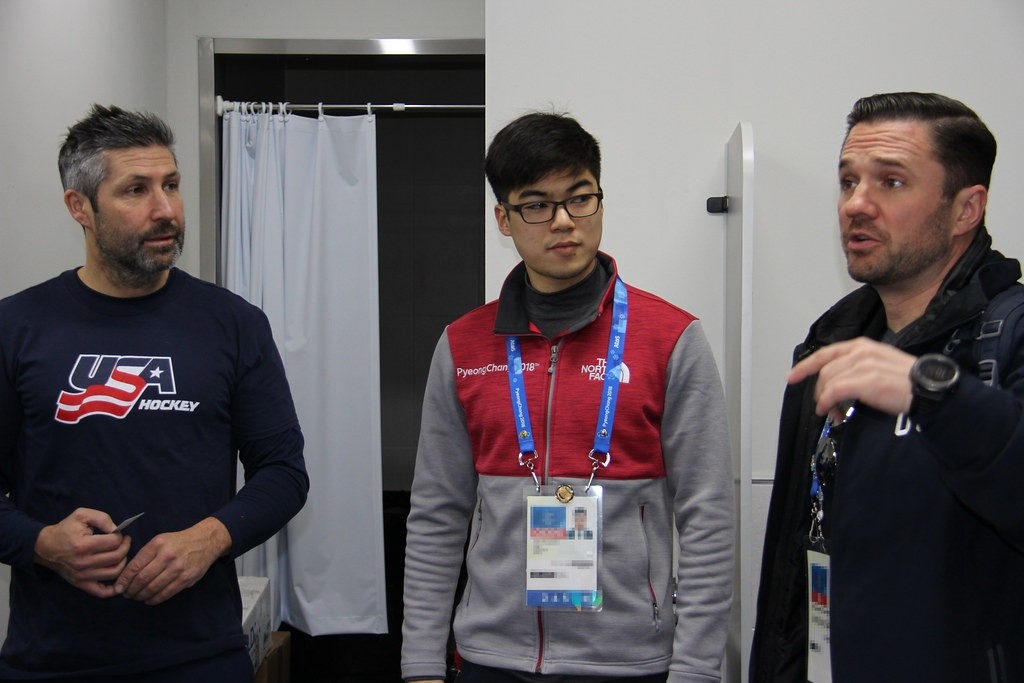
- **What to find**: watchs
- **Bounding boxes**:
[910,352,961,421]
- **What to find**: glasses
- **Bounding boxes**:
[501,186,604,224]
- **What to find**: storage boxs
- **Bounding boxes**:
[255,631,291,683]
[237,575,273,676]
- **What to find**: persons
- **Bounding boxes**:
[567,506,593,540]
[401,114,735,683]
[1,105,310,683]
[748,92,1024,683]
